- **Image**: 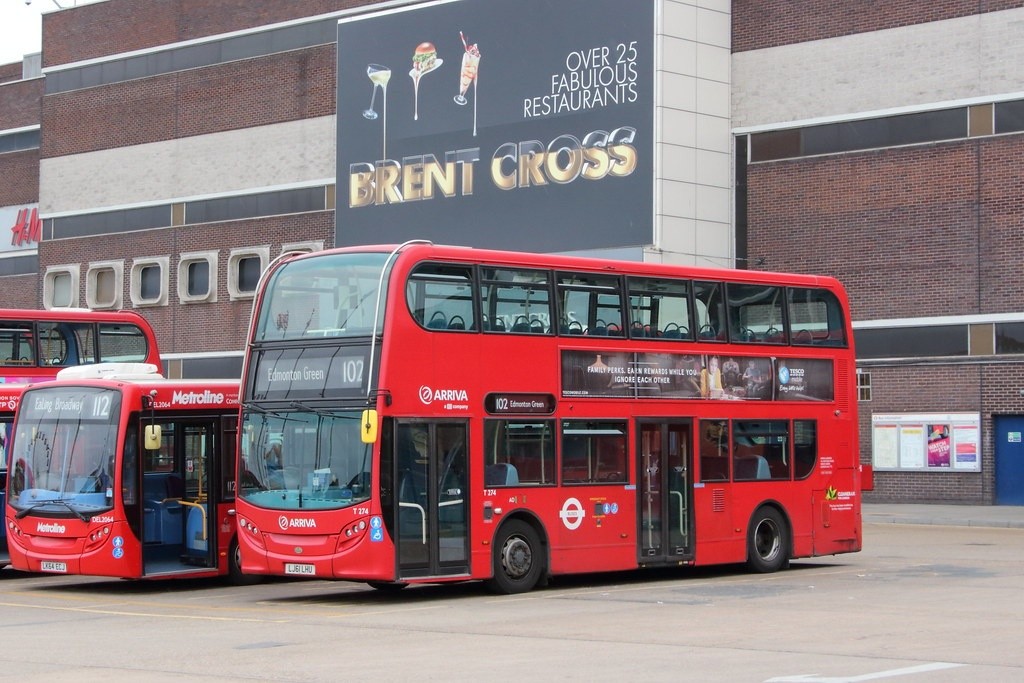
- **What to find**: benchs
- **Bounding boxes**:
[144,473,182,514]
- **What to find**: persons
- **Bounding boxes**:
[700,356,724,398]
[720,358,775,396]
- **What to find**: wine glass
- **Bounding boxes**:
[362,64,391,119]
[453,51,480,105]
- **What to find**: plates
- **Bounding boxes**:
[408,59,443,75]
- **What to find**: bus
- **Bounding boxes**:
[4,362,355,585]
[232,237,875,596]
[0,303,164,545]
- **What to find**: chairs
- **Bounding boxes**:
[568,321,582,335]
[592,319,608,336]
[737,325,749,341]
[678,326,689,339]
[426,310,447,329]
[793,329,813,344]
[631,321,645,337]
[486,464,519,486]
[469,314,490,331]
[699,324,716,340]
[607,323,621,336]
[493,318,506,332]
[764,328,781,342]
[662,322,681,338]
[530,319,544,334]
[741,329,756,342]
[447,316,465,329]
[546,318,570,334]
[510,315,531,332]
[734,454,771,480]
